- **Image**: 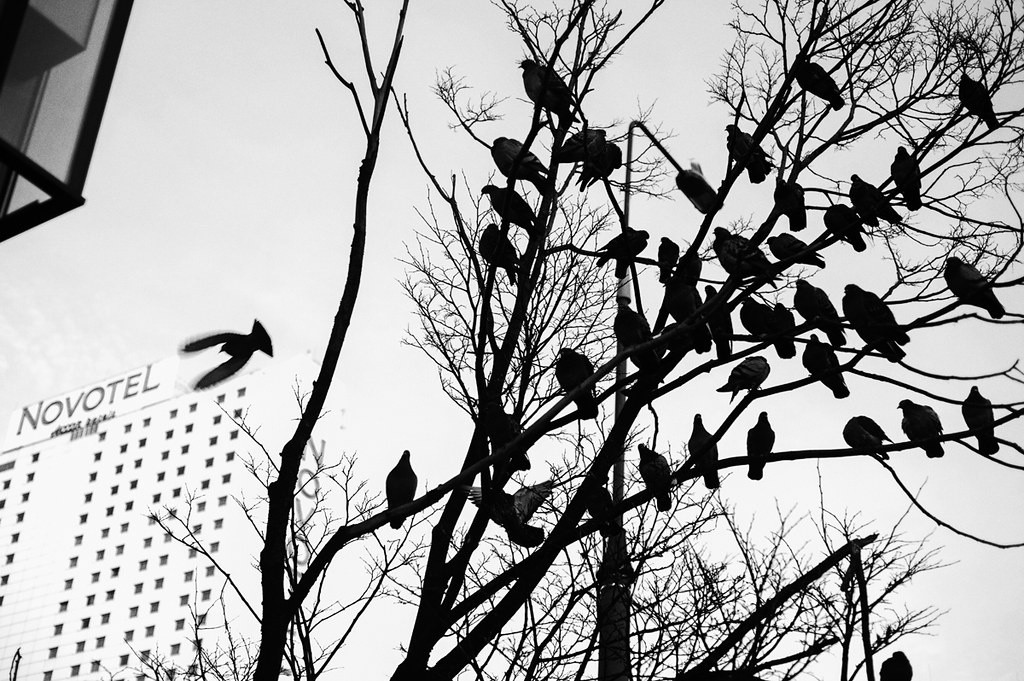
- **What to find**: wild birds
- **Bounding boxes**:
[180,319,274,392]
[843,415,893,464]
[596,227,649,268]
[467,479,557,548]
[688,414,722,488]
[491,137,560,196]
[745,412,776,481]
[962,386,1001,456]
[478,223,518,287]
[480,183,537,235]
[555,346,600,421]
[558,127,625,192]
[586,487,614,536]
[898,400,945,458]
[654,56,1010,399]
[611,305,664,382]
[637,443,671,512]
[879,651,915,681]
[386,451,419,529]
[520,58,582,124]
